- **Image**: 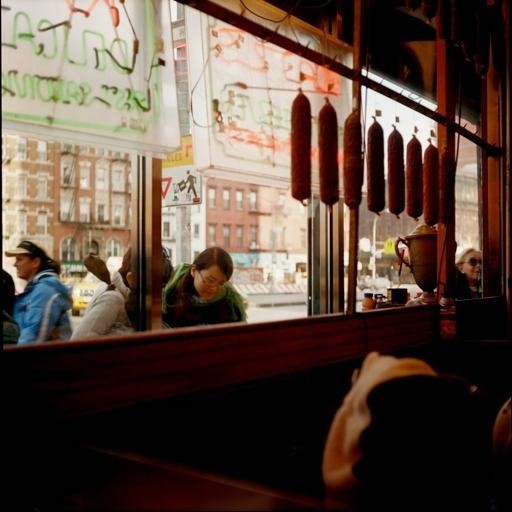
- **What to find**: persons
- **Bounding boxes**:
[68,243,172,340]
[1,267,19,349]
[5,239,73,347]
[456,248,482,299]
[160,246,247,327]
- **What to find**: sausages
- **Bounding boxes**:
[442,144,455,233]
[367,115,385,217]
[387,126,404,219]
[423,137,438,228]
[343,107,363,213]
[318,96,339,213]
[407,134,423,223]
[290,88,310,208]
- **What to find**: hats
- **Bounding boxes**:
[3,237,46,260]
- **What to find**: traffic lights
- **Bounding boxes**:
[213,99,222,125]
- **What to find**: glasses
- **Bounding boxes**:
[196,268,226,292]
[459,257,484,267]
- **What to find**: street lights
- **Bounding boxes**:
[371,207,391,279]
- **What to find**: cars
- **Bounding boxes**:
[62,277,84,291]
[70,255,126,318]
